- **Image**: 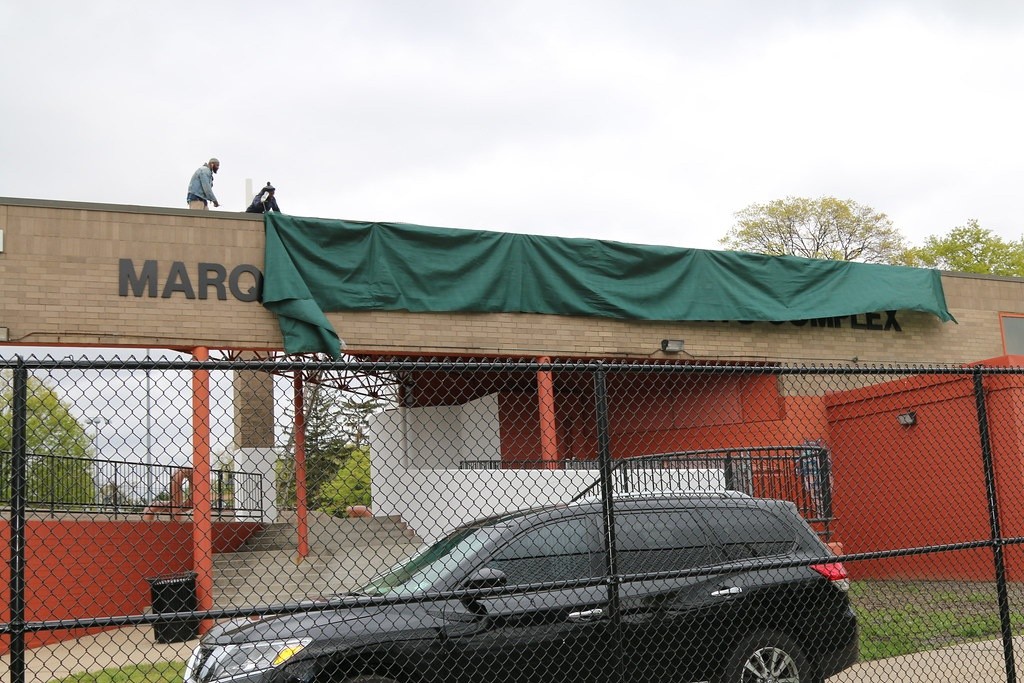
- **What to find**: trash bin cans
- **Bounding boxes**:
[145,571,198,643]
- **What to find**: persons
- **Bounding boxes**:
[245,182,280,213]
[187,158,219,210]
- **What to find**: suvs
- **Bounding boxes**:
[177,488,863,683]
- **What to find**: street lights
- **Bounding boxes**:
[85,415,111,505]
